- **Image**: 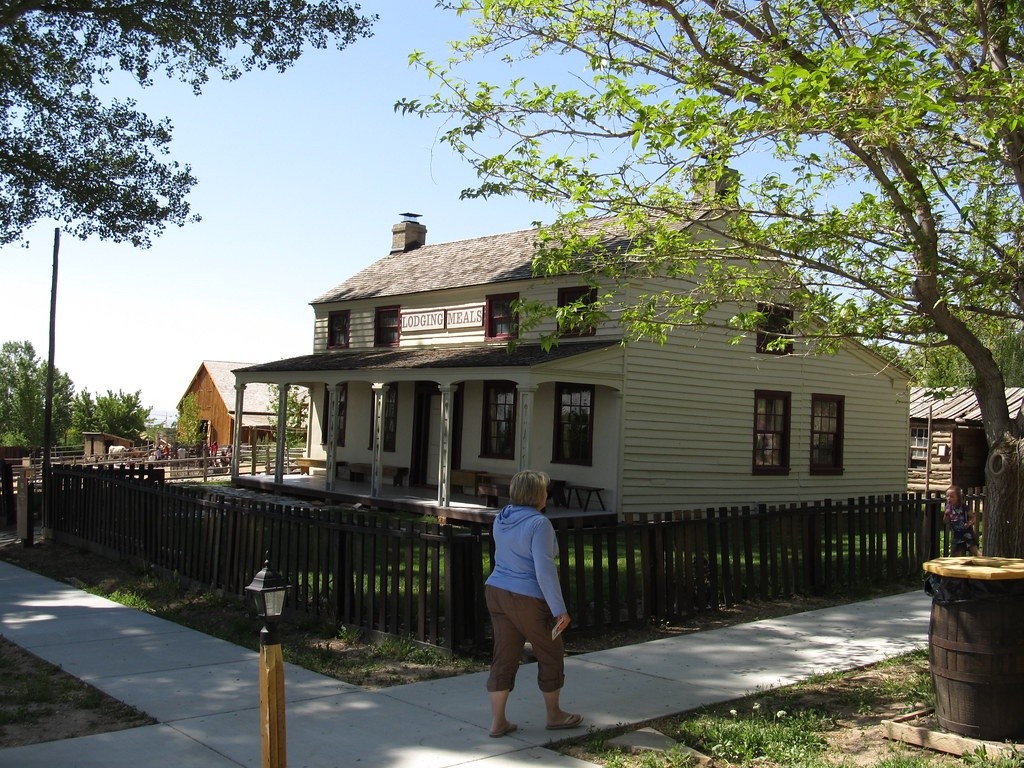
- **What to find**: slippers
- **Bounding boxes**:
[488,721,517,737]
[546,714,583,729]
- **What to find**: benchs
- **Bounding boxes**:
[564,486,606,512]
[296,458,347,478]
[476,473,568,515]
[451,468,487,497]
[348,463,409,487]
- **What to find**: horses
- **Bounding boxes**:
[108,445,145,460]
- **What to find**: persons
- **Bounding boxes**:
[126,447,140,458]
[220,444,233,474]
[210,442,218,463]
[943,486,982,557]
[485,470,584,738]
[95,453,100,462]
[195,445,201,465]
[155,446,169,466]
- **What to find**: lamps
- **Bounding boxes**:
[244,550,295,643]
